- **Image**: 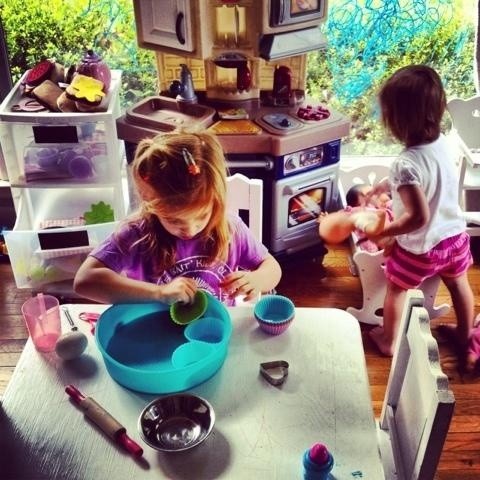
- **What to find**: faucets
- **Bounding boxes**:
[170,63,201,104]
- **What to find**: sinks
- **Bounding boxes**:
[126,96,213,130]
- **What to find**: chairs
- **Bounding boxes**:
[222,171,266,305]
[446,96,480,236]
[374,289,455,480]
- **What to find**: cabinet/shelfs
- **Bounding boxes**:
[0,69,129,297]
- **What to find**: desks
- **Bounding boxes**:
[0,304,386,479]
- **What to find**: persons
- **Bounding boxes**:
[318,207,396,249]
[346,183,372,206]
[364,66,475,357]
[73,131,281,306]
